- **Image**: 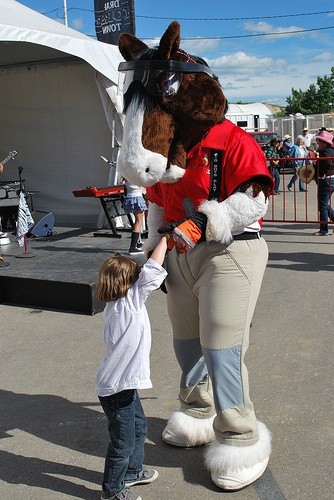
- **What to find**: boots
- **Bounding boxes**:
[129,232,142,252]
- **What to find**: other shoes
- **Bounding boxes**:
[287,185,292,192]
[300,190,307,192]
[271,191,280,195]
[312,231,325,236]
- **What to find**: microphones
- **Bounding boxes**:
[18,166,23,172]
[100,156,113,166]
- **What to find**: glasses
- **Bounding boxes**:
[303,130,307,132]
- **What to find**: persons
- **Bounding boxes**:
[123,179,149,255]
[266,137,282,195]
[311,130,334,236]
[287,134,312,192]
[276,127,334,174]
[95,230,173,500]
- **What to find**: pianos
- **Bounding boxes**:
[71,184,126,199]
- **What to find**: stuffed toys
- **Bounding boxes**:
[117,21,275,492]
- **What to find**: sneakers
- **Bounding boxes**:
[101,489,142,500]
[124,470,158,488]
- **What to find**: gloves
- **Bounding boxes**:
[147,250,168,294]
[157,211,207,254]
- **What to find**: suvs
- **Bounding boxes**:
[244,127,294,173]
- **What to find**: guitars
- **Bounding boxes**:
[0,149,18,169]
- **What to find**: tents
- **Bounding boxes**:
[0,0,146,233]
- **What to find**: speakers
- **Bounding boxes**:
[26,210,56,237]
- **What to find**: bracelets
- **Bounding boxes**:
[164,234,171,242]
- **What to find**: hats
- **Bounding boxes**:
[302,128,309,131]
[315,131,333,147]
[298,165,315,184]
[271,138,281,142]
[283,135,291,141]
[319,127,328,131]
